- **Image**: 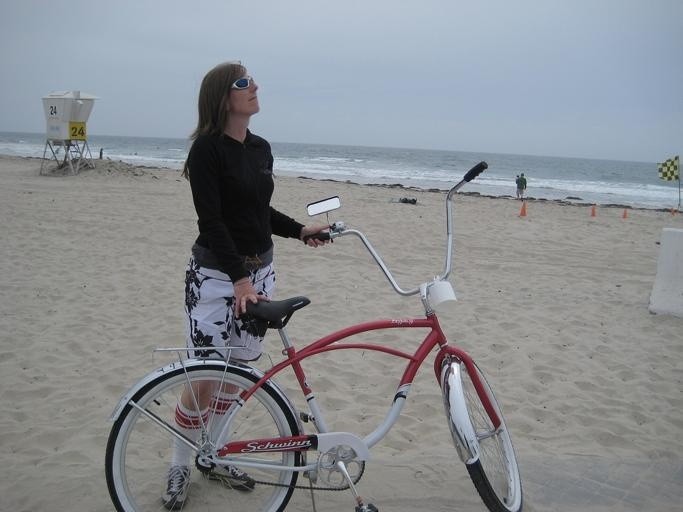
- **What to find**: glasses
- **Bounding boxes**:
[229,75,253,91]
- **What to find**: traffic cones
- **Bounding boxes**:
[591,204,597,216]
[624,209,627,218]
[520,199,528,216]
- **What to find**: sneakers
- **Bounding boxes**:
[202,457,256,491]
[161,463,191,511]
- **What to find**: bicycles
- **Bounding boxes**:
[105,162,523,512]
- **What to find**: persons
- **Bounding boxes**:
[161,63,331,510]
[516,173,526,199]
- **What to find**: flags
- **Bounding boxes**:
[657,155,679,182]
